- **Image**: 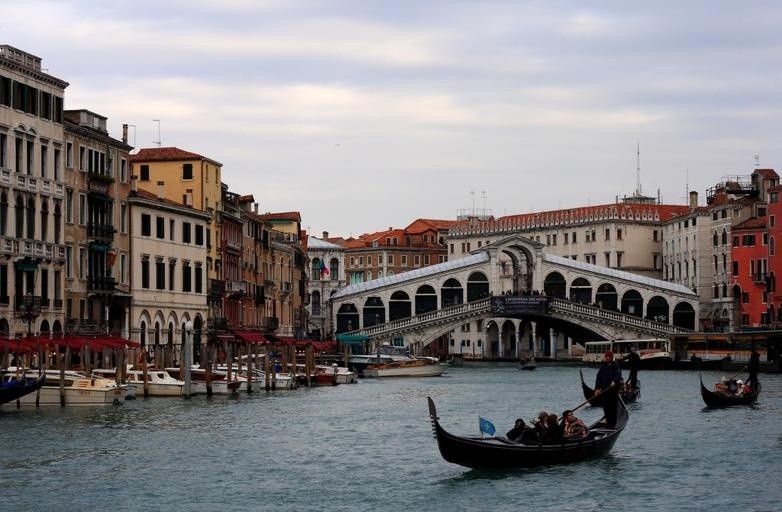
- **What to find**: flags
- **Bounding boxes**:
[107,250,117,266]
[319,258,329,277]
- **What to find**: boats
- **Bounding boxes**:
[700,365,762,409]
[427,369,641,470]
[518,357,537,371]
[583,330,782,370]
[0,346,448,408]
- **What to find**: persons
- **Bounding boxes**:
[735,380,752,397]
[482,288,600,308]
[745,347,760,392]
[506,410,588,445]
[595,351,620,430]
[620,348,641,392]
